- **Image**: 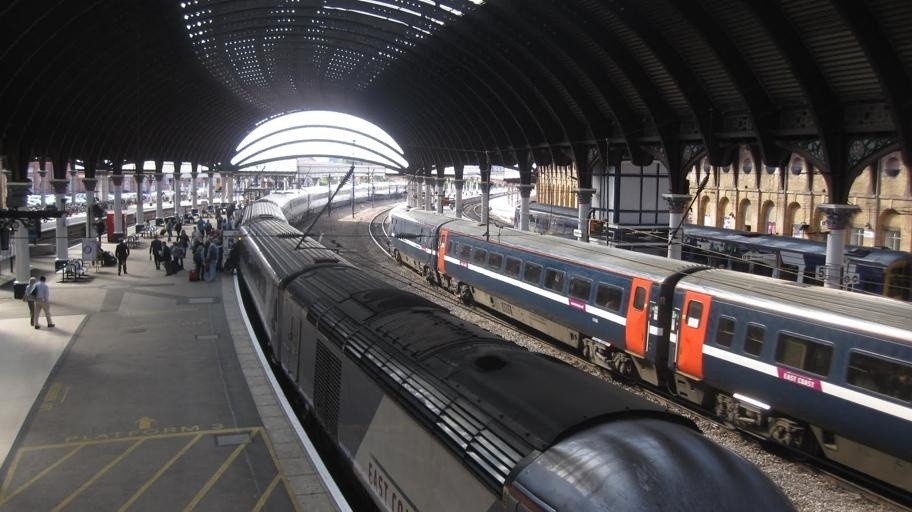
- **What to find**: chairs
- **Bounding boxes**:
[58,258,94,285]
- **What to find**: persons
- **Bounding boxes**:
[143,188,248,283]
[92,216,105,241]
[25,275,55,330]
[114,238,130,276]
